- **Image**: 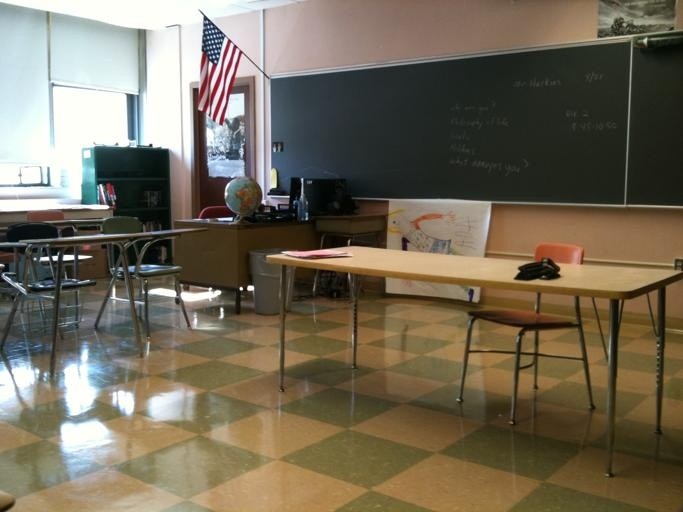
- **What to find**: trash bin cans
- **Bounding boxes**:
[250,249,295,315]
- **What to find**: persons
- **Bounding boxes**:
[224,116,243,159]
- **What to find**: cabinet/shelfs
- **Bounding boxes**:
[0,145,171,294]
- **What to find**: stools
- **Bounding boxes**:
[313,232,387,304]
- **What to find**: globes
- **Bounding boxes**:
[224,176,263,225]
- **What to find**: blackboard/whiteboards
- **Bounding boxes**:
[266,29,683,209]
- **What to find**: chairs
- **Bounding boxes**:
[454,243,596,427]
[199,206,237,219]
[0,216,192,378]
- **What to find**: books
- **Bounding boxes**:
[96,182,117,210]
[141,190,168,265]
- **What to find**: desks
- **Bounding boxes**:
[266,243,683,477]
[175,215,318,317]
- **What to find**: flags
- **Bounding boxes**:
[196,16,242,126]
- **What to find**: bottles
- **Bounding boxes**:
[292,176,309,221]
